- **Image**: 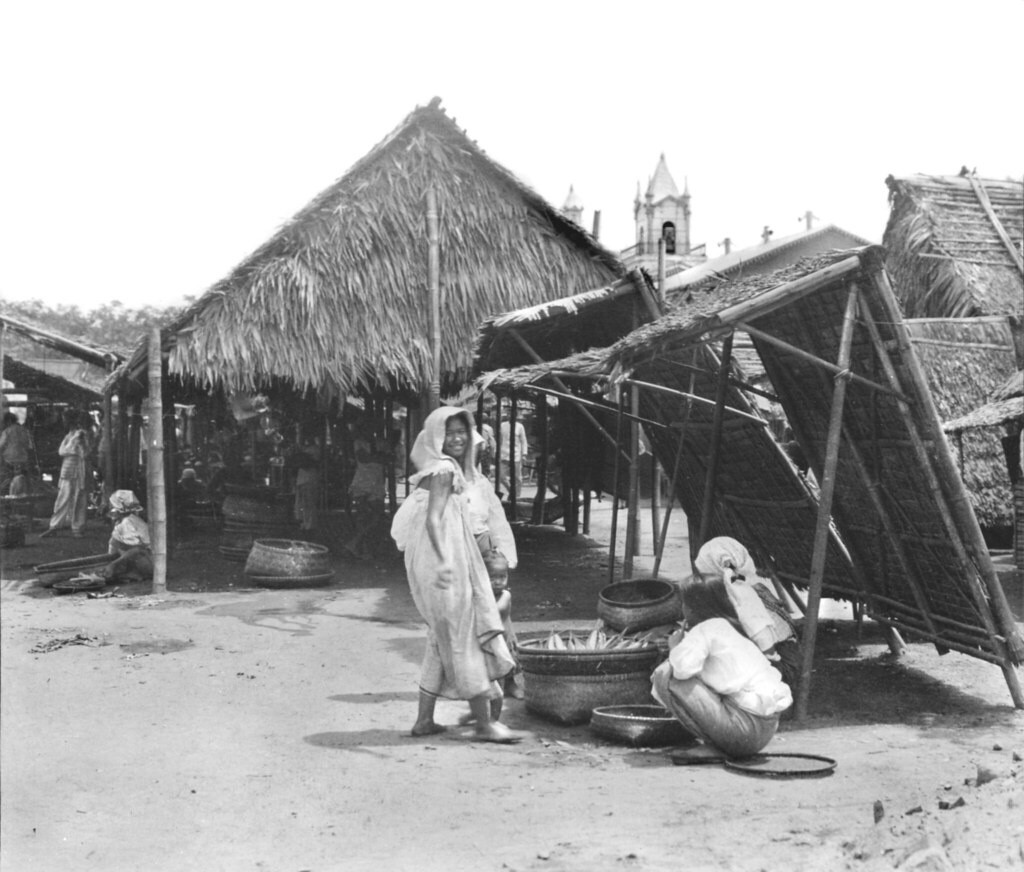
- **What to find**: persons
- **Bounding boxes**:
[390,406,518,741]
[340,412,396,559]
[137,416,149,470]
[176,442,227,513]
[97,489,153,584]
[695,535,805,690]
[0,405,104,539]
[460,552,513,730]
[476,413,496,478]
[500,407,528,502]
[287,425,322,540]
[649,574,793,765]
